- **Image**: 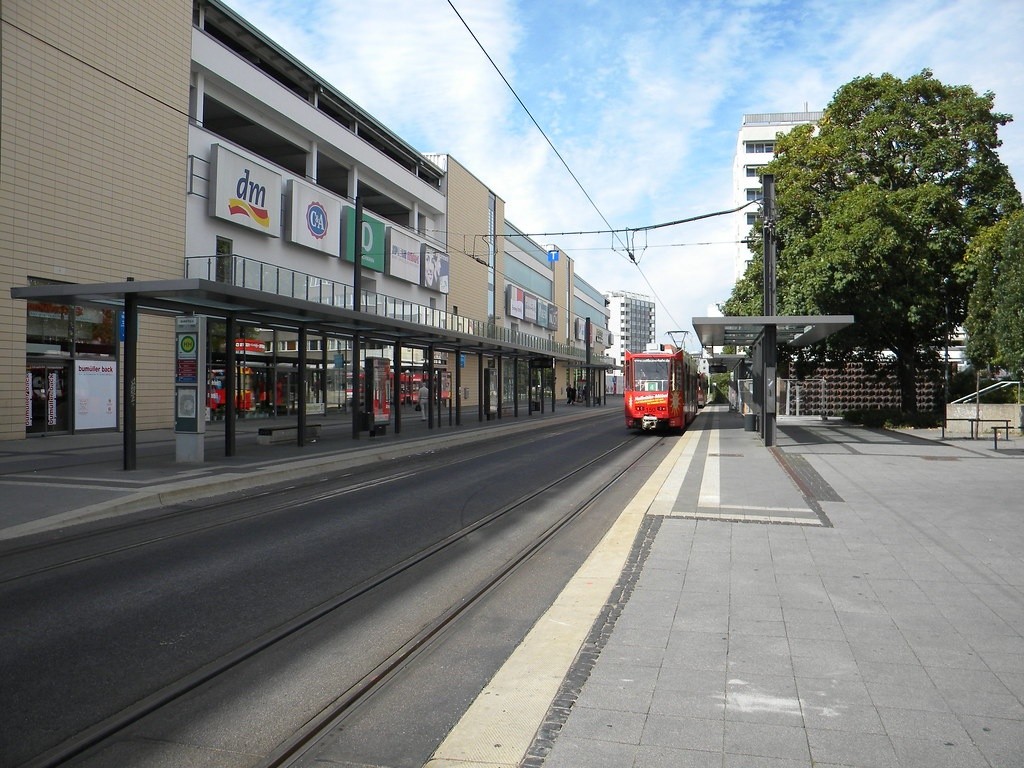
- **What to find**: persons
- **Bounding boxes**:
[566,385,587,405]
[417,382,429,421]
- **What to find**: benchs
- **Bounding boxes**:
[257,424,322,446]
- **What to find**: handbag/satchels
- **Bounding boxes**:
[415,405,421,411]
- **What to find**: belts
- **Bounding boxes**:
[420,398,428,399]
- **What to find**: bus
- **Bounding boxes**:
[695,369,710,409]
[622,344,702,436]
[346,369,452,405]
